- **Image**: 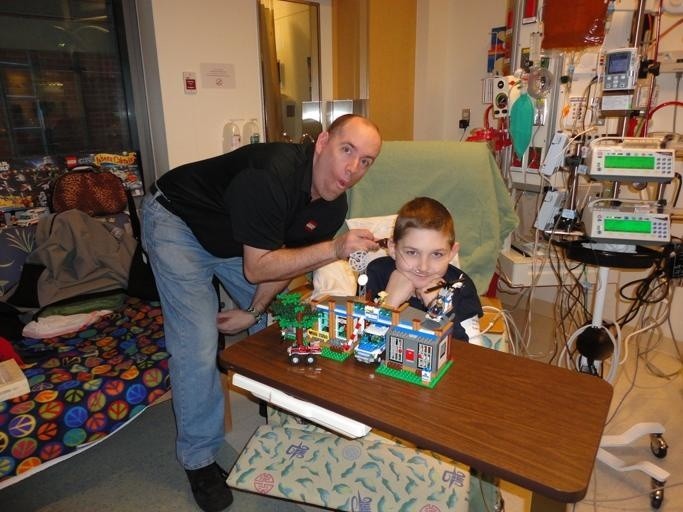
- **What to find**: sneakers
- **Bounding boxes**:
[185,459,233,512]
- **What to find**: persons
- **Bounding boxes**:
[137,113,380,512]
[365,196,484,348]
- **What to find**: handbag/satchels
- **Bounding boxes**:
[126,244,160,301]
[47,162,128,216]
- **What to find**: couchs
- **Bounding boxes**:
[1,217,172,491]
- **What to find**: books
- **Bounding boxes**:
[0,357,31,402]
[230,373,372,440]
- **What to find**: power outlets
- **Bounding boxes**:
[459,109,470,127]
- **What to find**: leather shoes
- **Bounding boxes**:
[149,181,181,216]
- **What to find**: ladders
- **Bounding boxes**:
[1,51,48,160]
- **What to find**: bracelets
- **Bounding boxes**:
[244,305,262,324]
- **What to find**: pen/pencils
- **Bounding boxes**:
[426,278,465,292]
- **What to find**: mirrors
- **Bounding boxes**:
[258,0,321,144]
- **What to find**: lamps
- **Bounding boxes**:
[299,101,321,122]
[327,100,360,123]
